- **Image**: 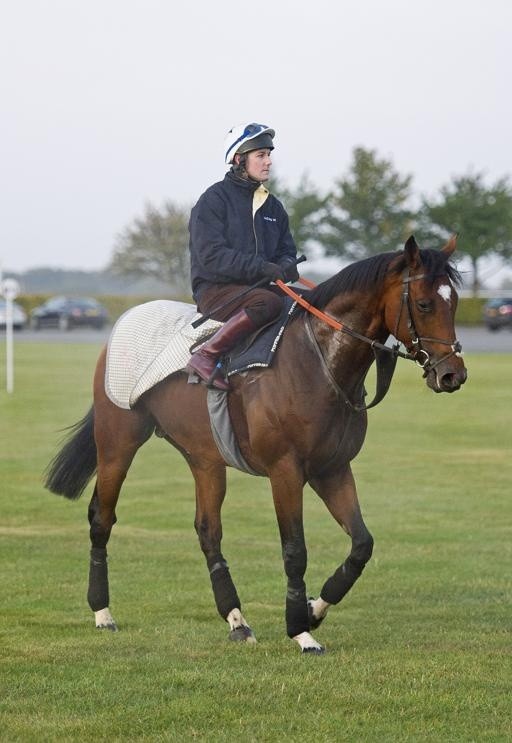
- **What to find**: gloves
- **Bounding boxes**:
[258,256,299,284]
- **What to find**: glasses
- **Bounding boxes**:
[232,123,269,162]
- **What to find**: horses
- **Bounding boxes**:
[38,230,470,653]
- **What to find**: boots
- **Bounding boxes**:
[189,309,256,390]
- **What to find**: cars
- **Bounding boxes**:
[0,296,107,330]
[480,297,510,328]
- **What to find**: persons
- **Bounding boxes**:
[188,122,297,390]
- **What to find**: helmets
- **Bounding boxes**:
[225,123,275,163]
